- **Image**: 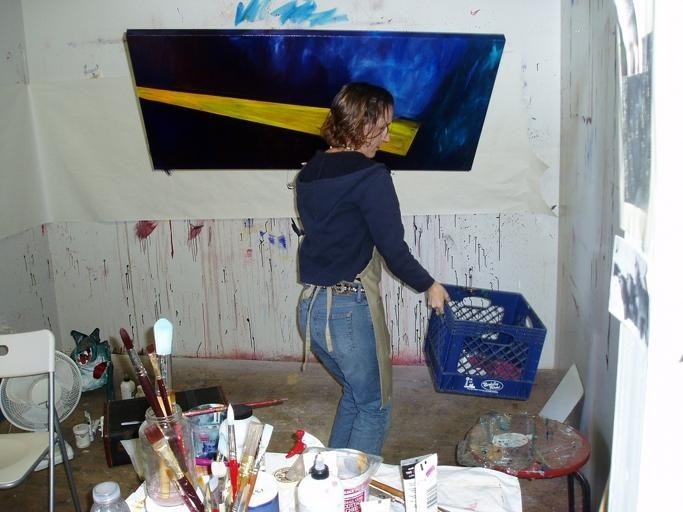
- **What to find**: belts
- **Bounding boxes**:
[309,283,365,296]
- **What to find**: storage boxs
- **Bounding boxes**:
[102,386,228,469]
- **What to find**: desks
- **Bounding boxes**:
[125,451,519,512]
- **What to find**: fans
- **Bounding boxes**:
[0,348,83,472]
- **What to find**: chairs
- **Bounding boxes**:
[0,329,81,512]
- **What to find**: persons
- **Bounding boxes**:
[294,82,450,456]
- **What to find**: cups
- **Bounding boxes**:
[313,448,369,512]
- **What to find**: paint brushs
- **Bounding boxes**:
[119,318,288,512]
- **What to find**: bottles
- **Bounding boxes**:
[119,376,145,400]
[90,400,344,512]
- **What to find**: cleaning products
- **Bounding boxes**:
[120,373,145,400]
[285,426,327,474]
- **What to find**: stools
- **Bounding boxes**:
[462,412,592,512]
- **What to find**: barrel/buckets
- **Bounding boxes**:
[325,446,373,509]
[73,423,91,448]
